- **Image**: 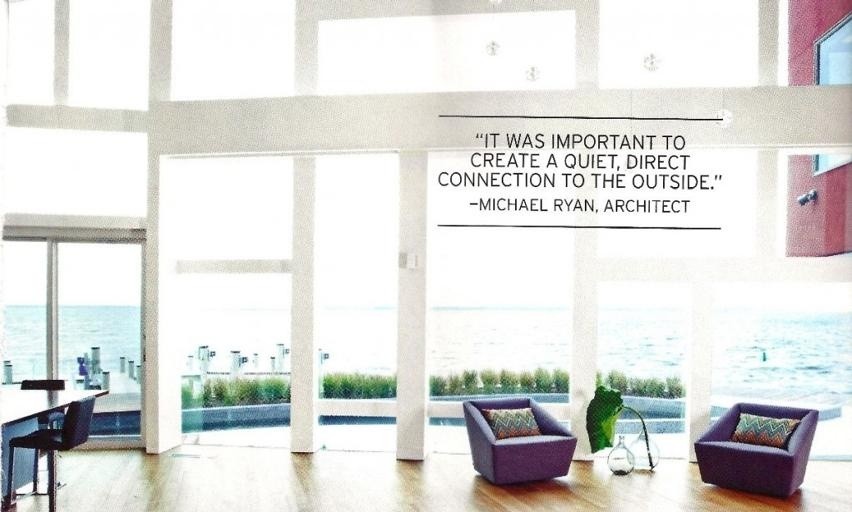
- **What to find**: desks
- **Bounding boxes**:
[1,387,108,501]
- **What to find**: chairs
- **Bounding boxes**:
[694,401,817,503]
[463,395,576,487]
[3,380,95,511]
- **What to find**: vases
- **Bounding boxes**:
[606,431,660,475]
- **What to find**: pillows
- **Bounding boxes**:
[479,406,542,442]
[727,408,797,451]
